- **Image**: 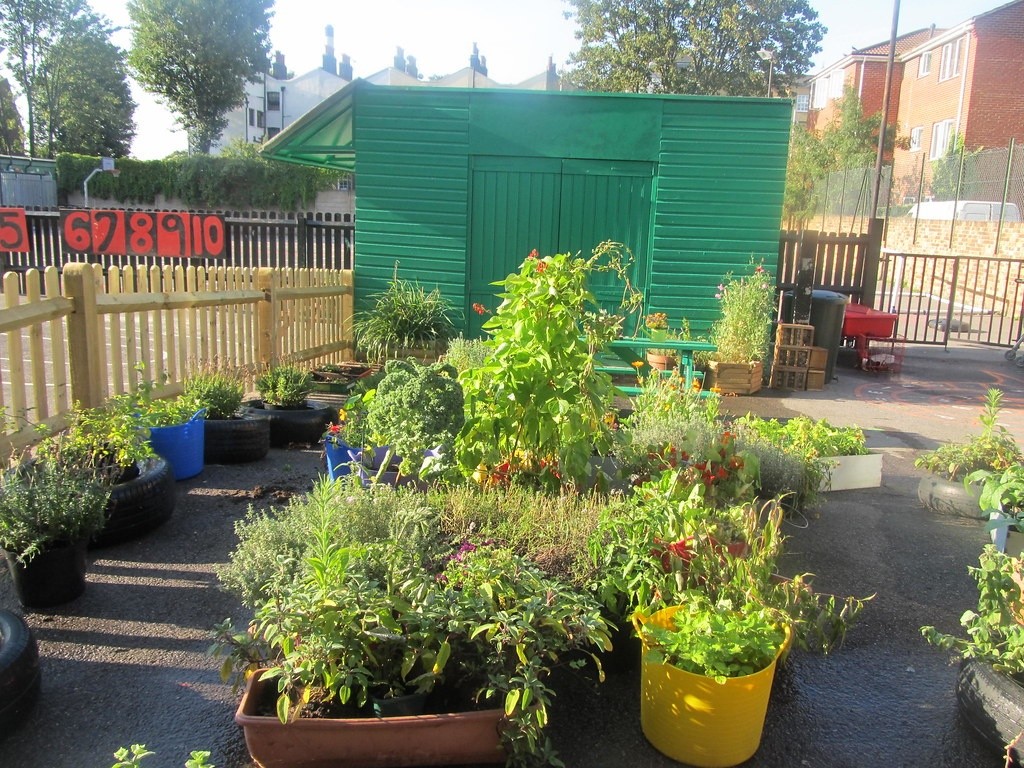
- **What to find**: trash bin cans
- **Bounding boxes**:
[780,290,849,383]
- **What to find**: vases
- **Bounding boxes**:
[323,434,363,481]
[703,361,762,395]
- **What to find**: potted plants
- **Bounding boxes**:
[0,239,1024,768]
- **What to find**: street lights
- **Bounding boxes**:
[757,50,778,96]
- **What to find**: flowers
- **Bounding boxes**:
[698,255,787,364]
[325,391,377,450]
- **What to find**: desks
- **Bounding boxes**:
[575,334,718,394]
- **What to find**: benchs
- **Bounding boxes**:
[613,384,716,399]
[593,365,704,380]
